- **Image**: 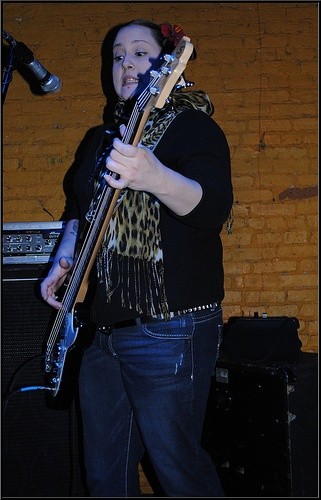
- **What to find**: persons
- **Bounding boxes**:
[41,21,233,497]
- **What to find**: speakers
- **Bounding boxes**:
[1,264,93,497]
[197,352,318,497]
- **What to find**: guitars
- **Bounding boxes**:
[42,35,195,412]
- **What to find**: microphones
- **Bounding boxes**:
[2,32,61,94]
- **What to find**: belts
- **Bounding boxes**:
[112,318,145,330]
